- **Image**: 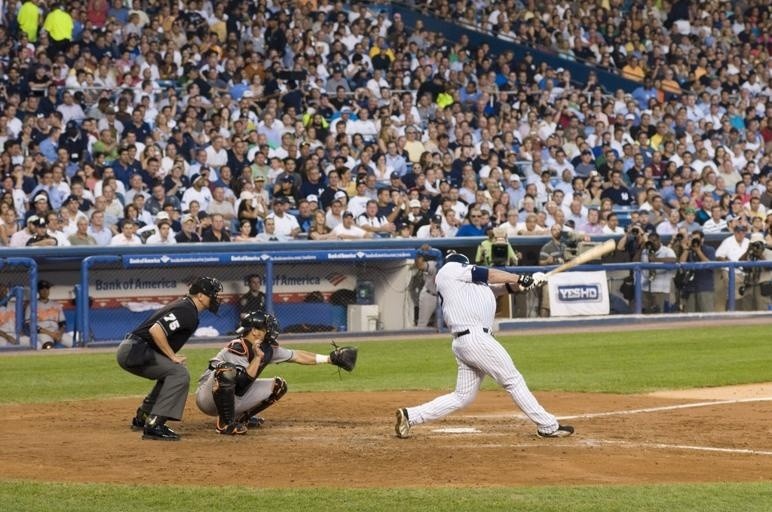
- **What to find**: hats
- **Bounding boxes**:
[38,280,53,289]
[735,225,745,231]
[442,253,470,265]
[510,174,520,182]
[726,214,742,222]
[157,211,171,220]
[750,234,766,245]
[432,215,442,224]
[237,176,353,219]
[398,223,409,228]
[191,173,202,185]
[391,171,399,179]
[409,199,422,208]
[181,215,195,224]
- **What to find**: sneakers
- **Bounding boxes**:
[536,425,574,439]
[395,408,410,438]
[133,408,180,441]
[236,416,265,426]
[216,424,248,434]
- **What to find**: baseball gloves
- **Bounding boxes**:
[330,347,356,371]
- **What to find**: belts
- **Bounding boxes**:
[426,290,438,297]
[458,328,488,337]
[125,333,144,342]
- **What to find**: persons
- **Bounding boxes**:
[395,252,574,439]
[195,310,357,435]
[0,0,772,349]
[117,275,224,441]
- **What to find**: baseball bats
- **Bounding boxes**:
[518,238,616,292]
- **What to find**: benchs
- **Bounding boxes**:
[25,302,336,352]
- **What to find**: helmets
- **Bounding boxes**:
[235,310,281,347]
[194,276,226,317]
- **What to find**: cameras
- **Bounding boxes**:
[752,240,764,251]
[691,238,700,247]
[632,228,639,235]
[677,234,683,241]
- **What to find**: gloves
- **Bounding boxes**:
[519,272,548,295]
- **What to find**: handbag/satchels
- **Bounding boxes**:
[620,284,635,299]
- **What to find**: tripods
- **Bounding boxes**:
[72,307,95,346]
